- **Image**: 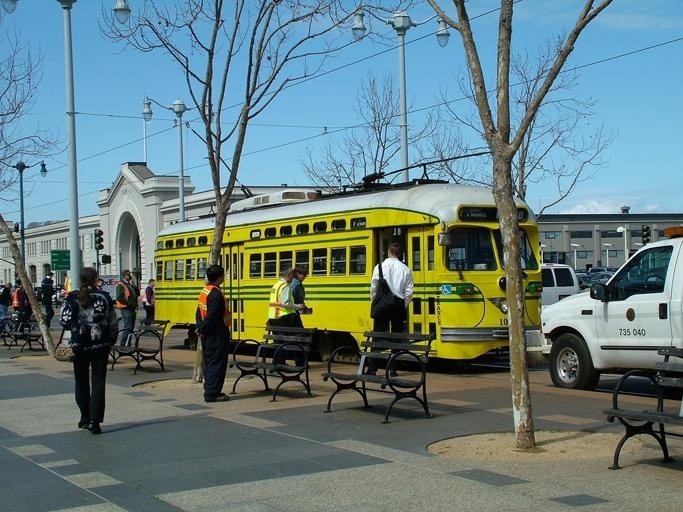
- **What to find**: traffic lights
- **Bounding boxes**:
[93,229,103,250]
[14,223,18,233]
[101,255,111,264]
[641,225,650,246]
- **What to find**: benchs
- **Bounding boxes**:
[228,323,318,407]
[0,311,52,353]
[598,344,683,473]
[317,327,438,431]
[108,318,173,378]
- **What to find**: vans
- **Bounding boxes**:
[539,262,581,305]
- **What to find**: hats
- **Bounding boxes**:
[119,269,132,276]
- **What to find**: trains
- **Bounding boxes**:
[152,148,544,370]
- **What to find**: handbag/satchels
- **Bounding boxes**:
[54,344,80,363]
[371,279,395,319]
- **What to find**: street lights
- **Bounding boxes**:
[0,160,48,267]
[540,243,545,264]
[1,0,132,361]
[569,242,580,270]
[602,242,613,267]
[633,242,643,250]
[350,3,450,183]
[142,95,215,222]
[616,226,628,262]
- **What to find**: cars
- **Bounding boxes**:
[0,283,66,306]
[540,225,682,390]
[574,266,619,290]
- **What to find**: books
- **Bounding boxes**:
[298,307,312,314]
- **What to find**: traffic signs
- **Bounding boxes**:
[50,249,83,271]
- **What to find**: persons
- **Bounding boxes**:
[58,266,119,434]
[0,269,155,347]
[194,263,232,402]
[267,266,309,369]
[366,243,413,377]
[288,264,309,329]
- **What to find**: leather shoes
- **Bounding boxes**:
[220,391,225,395]
[390,369,399,376]
[87,420,102,434]
[78,418,89,428]
[366,370,377,377]
[216,396,230,402]
